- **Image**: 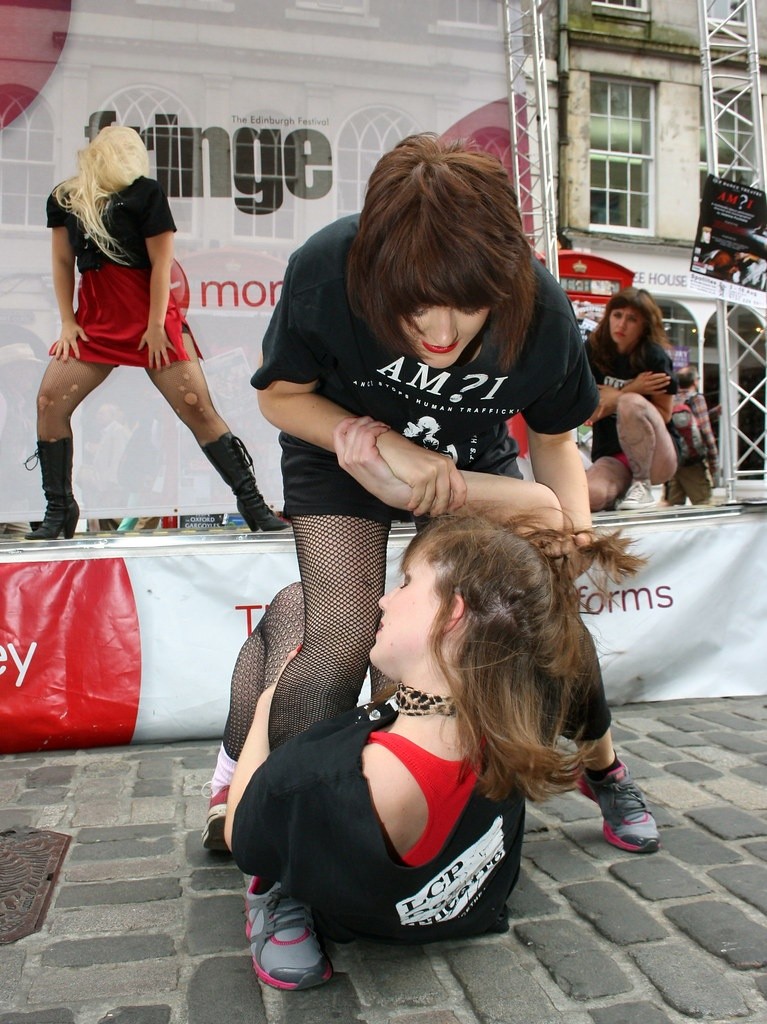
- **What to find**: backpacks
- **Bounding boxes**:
[668,393,711,465]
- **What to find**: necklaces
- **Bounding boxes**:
[395,681,458,718]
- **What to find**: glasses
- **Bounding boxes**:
[696,376,700,379]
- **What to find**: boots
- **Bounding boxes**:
[200,432,289,532]
[22,436,80,539]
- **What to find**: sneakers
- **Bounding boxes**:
[573,755,660,852]
[200,778,233,850]
[244,874,335,991]
[612,479,658,509]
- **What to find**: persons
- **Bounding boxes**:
[202,415,645,943]
[659,366,720,507]
[246,132,659,990]
[583,288,681,510]
[24,126,289,540]
